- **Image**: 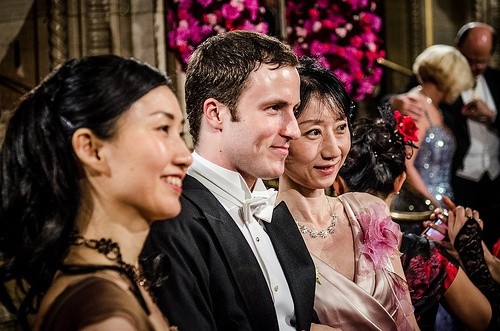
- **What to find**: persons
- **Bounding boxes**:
[333,104,492,331]
[391,44,474,235]
[138,30,316,331]
[274,56,419,331]
[382,22,500,254]
[0,54,193,331]
[424,195,500,284]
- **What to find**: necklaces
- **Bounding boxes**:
[297,196,337,239]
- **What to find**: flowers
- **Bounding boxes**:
[167,0,388,102]
[355,203,403,269]
[394,111,420,144]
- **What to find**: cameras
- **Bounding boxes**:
[422,212,451,245]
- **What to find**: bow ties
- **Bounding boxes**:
[238,188,278,225]
